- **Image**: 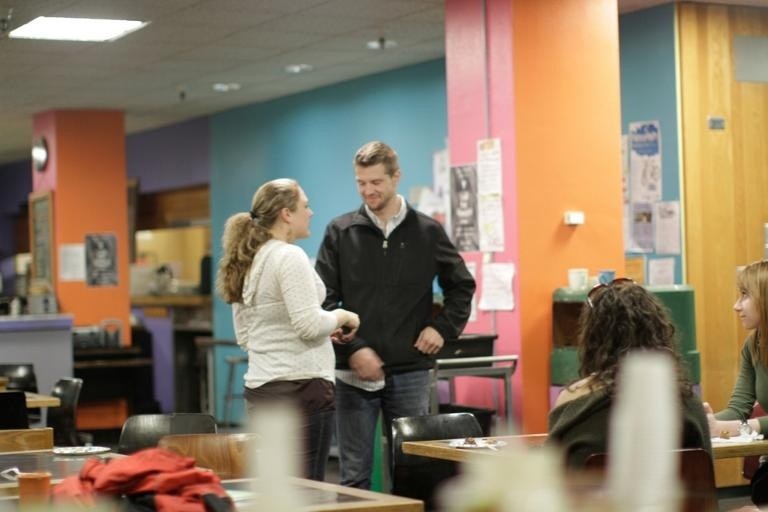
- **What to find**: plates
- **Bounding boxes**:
[52,445,111,455]
[450,437,506,449]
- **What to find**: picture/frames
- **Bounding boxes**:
[28,188,57,291]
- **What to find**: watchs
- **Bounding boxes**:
[738,416,752,437]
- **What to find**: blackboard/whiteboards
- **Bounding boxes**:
[27,190,54,293]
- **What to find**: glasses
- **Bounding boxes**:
[587,278,632,308]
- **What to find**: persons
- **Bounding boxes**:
[543,276,719,510]
[216,179,362,478]
[313,140,478,492]
[704,256,768,510]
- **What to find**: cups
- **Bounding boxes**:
[599,269,615,287]
[567,268,589,294]
[17,472,52,512]
